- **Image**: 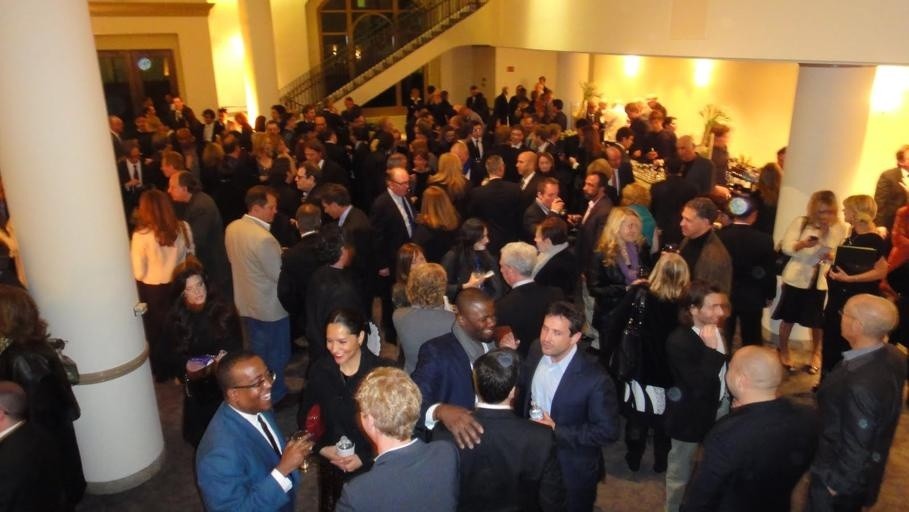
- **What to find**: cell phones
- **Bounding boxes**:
[810,235,818,241]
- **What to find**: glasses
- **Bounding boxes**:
[230,368,278,390]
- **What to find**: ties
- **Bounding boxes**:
[133,164,140,180]
[475,140,481,159]
[257,414,282,458]
[402,197,415,231]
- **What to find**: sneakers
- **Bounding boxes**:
[624,453,667,475]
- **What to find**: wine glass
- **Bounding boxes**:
[291,428,313,472]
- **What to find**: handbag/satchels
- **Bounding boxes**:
[171,254,204,282]
[775,241,794,276]
[618,316,660,365]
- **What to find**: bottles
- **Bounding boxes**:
[335,435,356,455]
[529,400,543,419]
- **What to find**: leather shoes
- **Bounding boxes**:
[807,364,819,375]
[775,346,797,373]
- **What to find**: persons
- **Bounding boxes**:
[109,76,908,509]
[0,192,87,511]
